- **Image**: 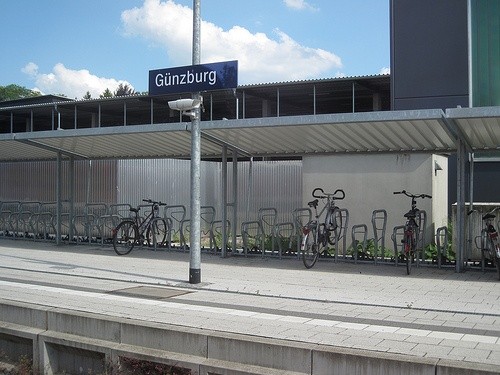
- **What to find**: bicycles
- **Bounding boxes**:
[392,189,433,275]
[467,206,500,280]
[300,188,345,269]
[111,198,168,255]
